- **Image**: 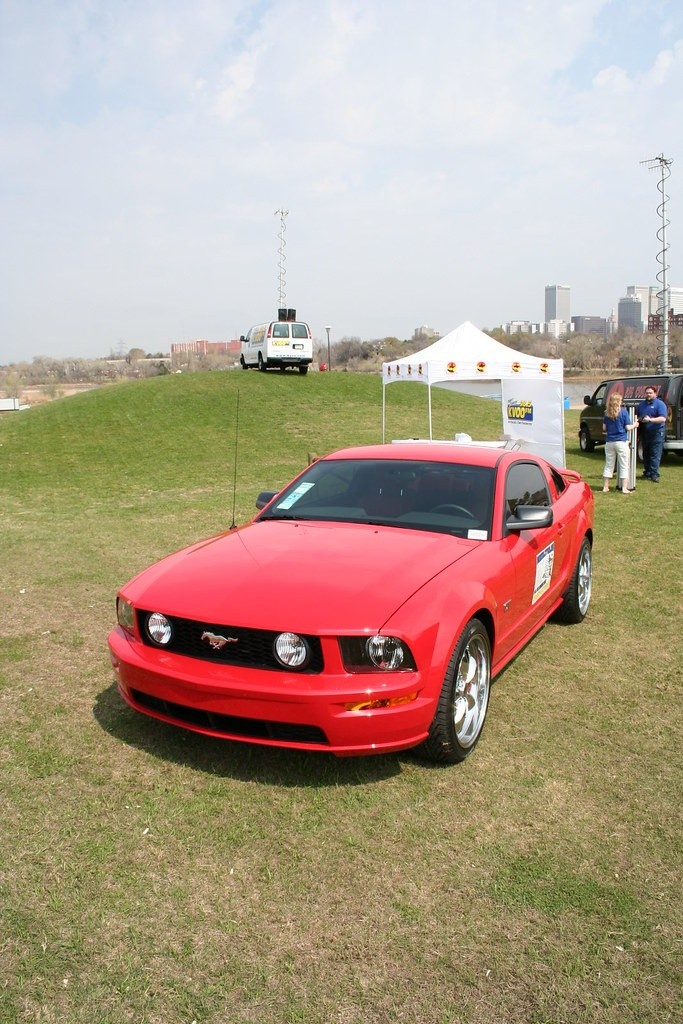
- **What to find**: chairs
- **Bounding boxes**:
[360,472,493,521]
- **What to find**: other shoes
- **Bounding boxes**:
[651,475,660,483]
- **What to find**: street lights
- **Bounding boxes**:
[325,326,331,373]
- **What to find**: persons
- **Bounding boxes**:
[636,386,668,483]
[602,392,639,494]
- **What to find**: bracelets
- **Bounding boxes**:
[649,418,651,423]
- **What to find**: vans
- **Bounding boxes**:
[239,321,313,375]
[578,375,683,463]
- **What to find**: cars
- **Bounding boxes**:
[107,444,595,769]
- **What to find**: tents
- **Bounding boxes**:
[382,321,566,470]
